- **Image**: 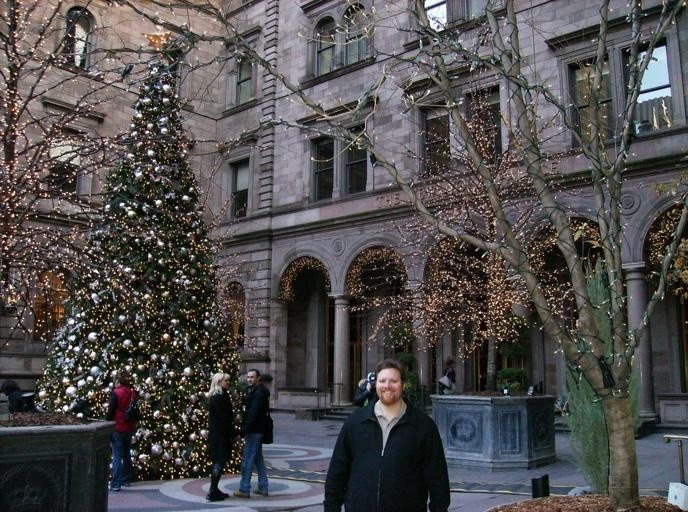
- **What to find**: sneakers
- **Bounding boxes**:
[254,488,268,496]
[217,490,229,498]
[108,484,120,491]
[233,490,250,498]
[206,492,224,501]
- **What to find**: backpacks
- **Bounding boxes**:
[118,389,140,425]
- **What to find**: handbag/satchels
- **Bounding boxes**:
[437,369,454,389]
[263,415,273,444]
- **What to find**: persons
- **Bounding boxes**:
[108,372,138,491]
[353,372,377,409]
[438,359,456,395]
[320,357,452,512]
[232,367,270,498]
[205,372,236,501]
[2,380,41,413]
